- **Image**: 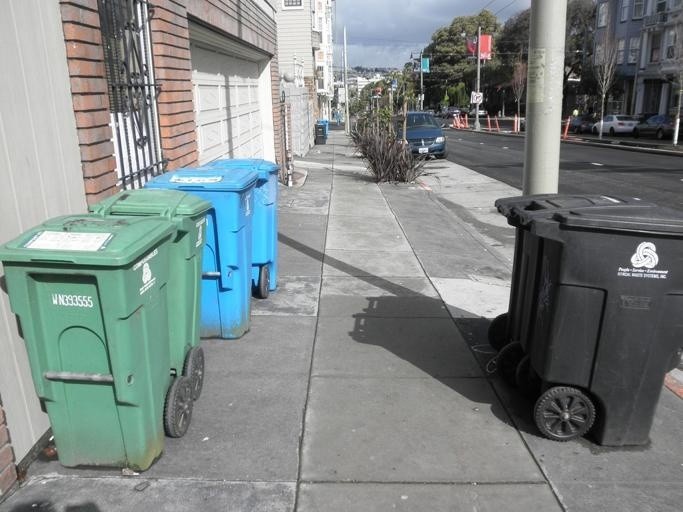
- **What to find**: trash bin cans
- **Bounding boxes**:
[143,167,257,340]
[0,214,178,471]
[487,193,683,447]
[315,119,328,145]
[203,157,280,298]
[92,189,215,399]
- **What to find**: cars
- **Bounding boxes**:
[443,104,488,119]
[561,113,683,139]
[392,111,448,159]
